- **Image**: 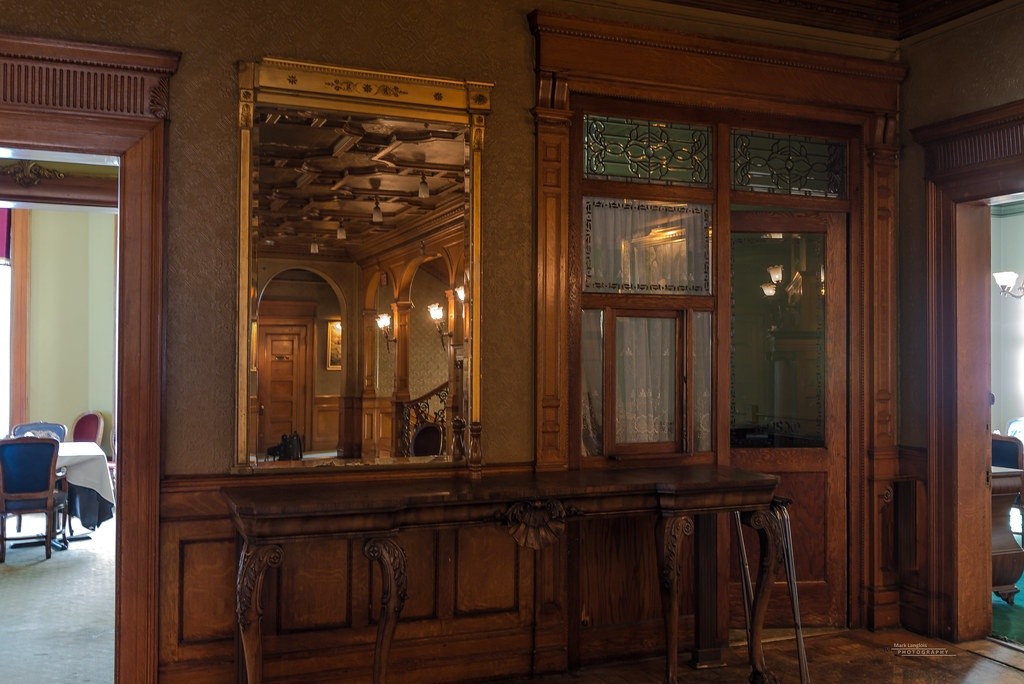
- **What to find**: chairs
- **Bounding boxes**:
[992,435,1024,549]
[0,410,114,563]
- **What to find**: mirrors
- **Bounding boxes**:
[247,99,470,462]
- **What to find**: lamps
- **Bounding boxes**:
[377,286,465,351]
[760,265,802,311]
[994,270,1024,298]
[309,176,428,253]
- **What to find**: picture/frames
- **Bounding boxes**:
[327,317,344,370]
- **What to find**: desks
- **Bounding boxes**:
[11,442,115,551]
[223,463,809,684]
